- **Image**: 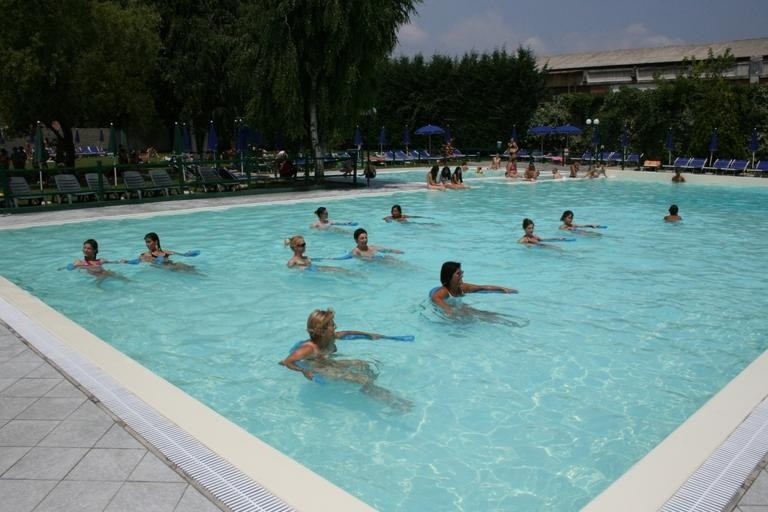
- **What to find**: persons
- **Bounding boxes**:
[364,161,376,178]
[340,160,352,176]
[288,206,434,275]
[519,217,570,253]
[123,231,200,277]
[559,210,598,236]
[57,238,130,286]
[672,166,684,183]
[665,205,681,222]
[281,309,413,408]
[119,144,160,163]
[2,139,54,176]
[279,157,298,181]
[427,138,606,189]
[434,260,529,331]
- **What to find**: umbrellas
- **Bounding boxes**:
[183,123,191,161]
[99,127,105,152]
[209,120,219,169]
[354,123,451,151]
[74,128,80,153]
[236,126,246,175]
[33,121,47,190]
[511,122,718,156]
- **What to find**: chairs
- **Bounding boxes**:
[490,146,768,178]
[74,146,106,158]
[294,148,465,167]
[0,165,269,209]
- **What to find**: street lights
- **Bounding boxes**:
[583,119,600,171]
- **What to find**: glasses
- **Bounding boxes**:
[298,243,305,246]
